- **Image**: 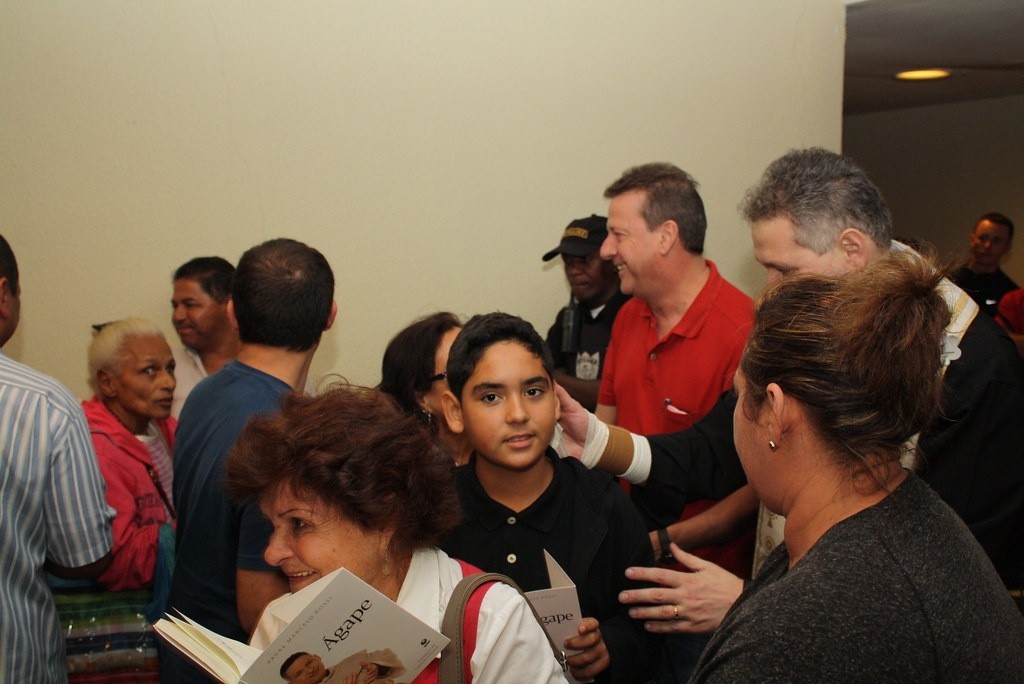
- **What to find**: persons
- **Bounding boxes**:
[432,313,679,684]
[82,317,178,592]
[547,147,1023,633]
[682,250,1024,684]
[227,387,569,684]
[382,311,472,466]
[947,214,1024,356]
[593,163,757,574]
[280,648,406,684]
[0,235,118,684]
[164,238,338,684]
[171,257,241,422]
[540,214,634,414]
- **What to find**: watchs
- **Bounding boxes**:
[656,528,677,566]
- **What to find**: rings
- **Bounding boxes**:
[673,605,679,619]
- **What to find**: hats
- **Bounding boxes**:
[542,214,607,261]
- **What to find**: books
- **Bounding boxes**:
[523,549,595,684]
[151,568,452,684]
